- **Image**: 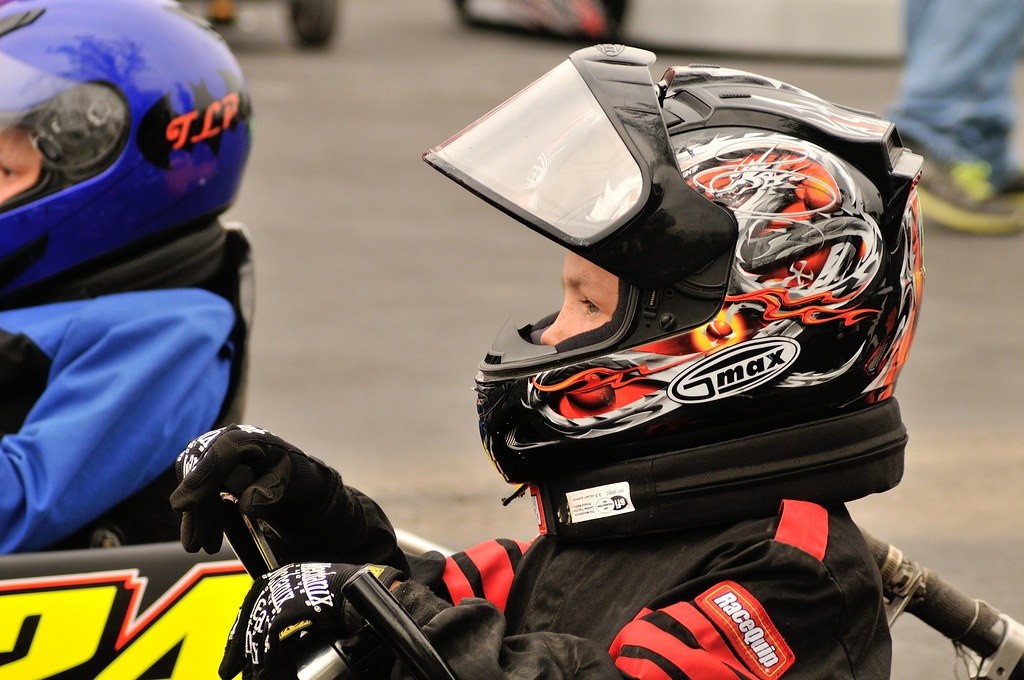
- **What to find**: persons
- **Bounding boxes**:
[172,43,924,679]
[885,0,1024,236]
[1,0,253,557]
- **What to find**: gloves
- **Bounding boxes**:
[171,424,366,558]
[218,561,405,680]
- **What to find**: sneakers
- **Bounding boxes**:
[908,146,1024,232]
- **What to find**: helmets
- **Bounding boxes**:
[475,67,927,538]
[0,0,256,300]
